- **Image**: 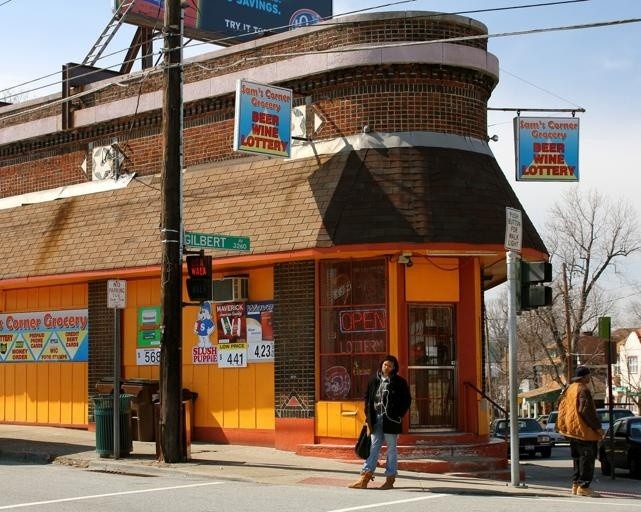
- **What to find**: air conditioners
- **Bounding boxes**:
[212,277,249,304]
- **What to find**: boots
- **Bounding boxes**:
[378,471,397,490]
[348,471,372,489]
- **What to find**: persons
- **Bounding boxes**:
[348,355,412,489]
[557,366,605,497]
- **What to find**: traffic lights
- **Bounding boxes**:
[186,254,216,303]
[523,259,554,312]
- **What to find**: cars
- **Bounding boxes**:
[492,408,641,479]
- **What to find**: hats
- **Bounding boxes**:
[572,366,590,381]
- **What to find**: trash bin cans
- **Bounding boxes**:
[152,392,197,461]
[90,394,134,459]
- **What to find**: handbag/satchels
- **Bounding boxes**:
[354,424,371,460]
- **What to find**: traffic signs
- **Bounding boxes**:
[184,230,250,253]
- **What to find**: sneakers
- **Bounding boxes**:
[571,483,600,497]
